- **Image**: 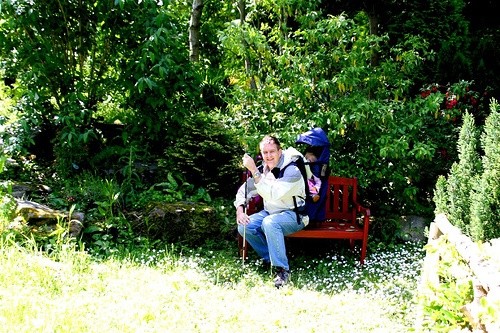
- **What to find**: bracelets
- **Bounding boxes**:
[252,169,260,177]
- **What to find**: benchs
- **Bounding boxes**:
[237,171,371,267]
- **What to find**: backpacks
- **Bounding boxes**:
[294,127,331,223]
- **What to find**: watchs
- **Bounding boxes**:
[253,172,261,180]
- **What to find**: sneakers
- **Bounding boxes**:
[274,267,291,287]
[262,261,270,271]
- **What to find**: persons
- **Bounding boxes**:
[232,134,312,287]
[304,152,322,198]
